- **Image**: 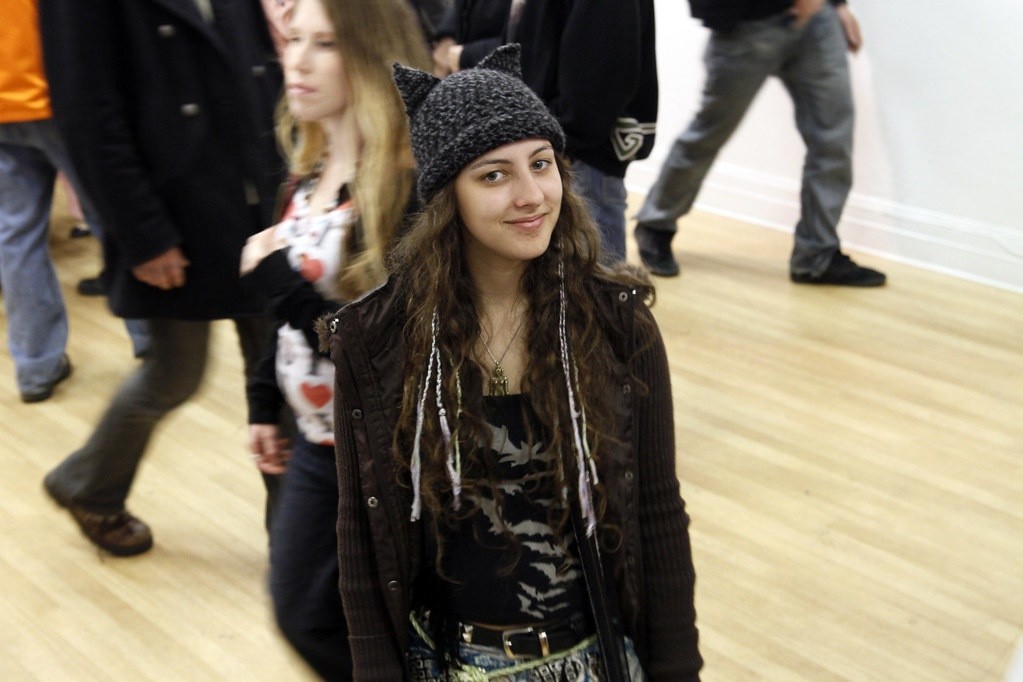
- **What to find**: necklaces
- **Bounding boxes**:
[477,319,524,395]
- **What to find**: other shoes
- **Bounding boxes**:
[70,222,92,237]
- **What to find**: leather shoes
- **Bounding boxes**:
[22,351,71,403]
[77,277,106,296]
[46,473,153,556]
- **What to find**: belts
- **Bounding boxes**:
[414,606,595,662]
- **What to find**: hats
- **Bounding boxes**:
[394,41,566,204]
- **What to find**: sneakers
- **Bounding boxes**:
[791,250,885,286]
[638,245,679,277]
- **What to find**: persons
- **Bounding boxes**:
[632,0,887,286]
[314,42,705,682]
[0,0,660,682]
